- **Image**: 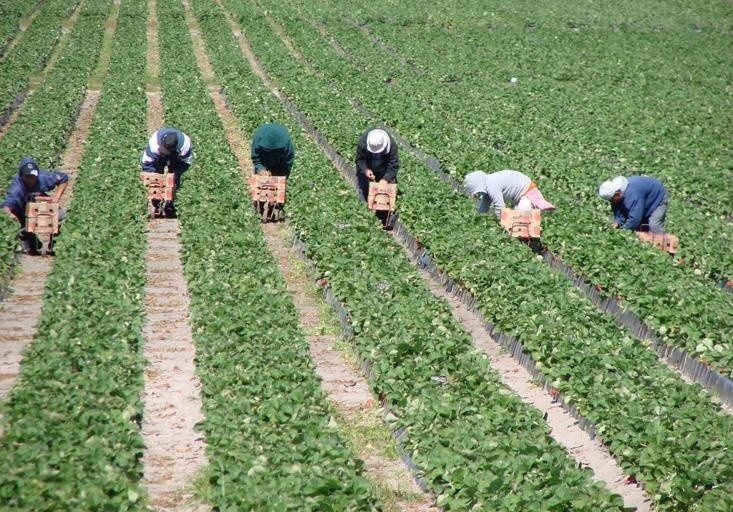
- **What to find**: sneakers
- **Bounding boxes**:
[154,205,178,219]
[26,237,57,255]
[258,208,276,221]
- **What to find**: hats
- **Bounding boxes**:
[366,128,391,156]
[159,132,178,156]
[21,162,39,176]
[599,177,624,201]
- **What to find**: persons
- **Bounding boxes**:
[139,126,193,218]
[598,175,668,235]
[2,158,68,257]
[355,128,399,210]
[250,122,294,222]
[463,170,555,252]
